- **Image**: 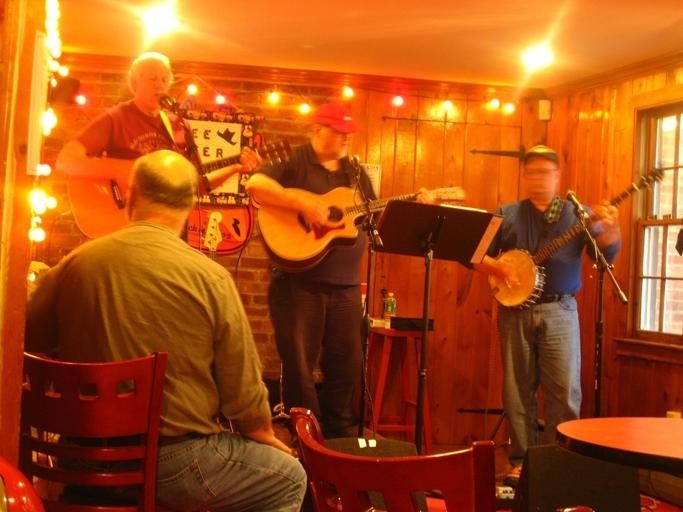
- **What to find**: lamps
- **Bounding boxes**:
[19,349,168,511]
[288,408,497,511]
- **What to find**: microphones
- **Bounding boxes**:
[368,328,433,455]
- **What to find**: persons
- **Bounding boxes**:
[245,102,440,439]
[464,142,624,487]
[53,50,264,245]
[24,150,307,512]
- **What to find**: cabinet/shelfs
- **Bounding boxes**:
[383,291,397,329]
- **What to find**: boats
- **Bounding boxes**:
[510,445,642,512]
[325,438,428,512]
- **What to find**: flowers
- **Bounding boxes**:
[337,148,357,189]
[157,93,185,117]
[567,191,591,227]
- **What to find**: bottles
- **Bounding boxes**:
[305,103,362,134]
[523,144,559,165]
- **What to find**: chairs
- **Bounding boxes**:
[503,464,521,486]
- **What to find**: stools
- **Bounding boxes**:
[128,435,199,445]
[537,292,575,302]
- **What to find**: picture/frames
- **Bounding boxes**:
[556,417,682,479]
